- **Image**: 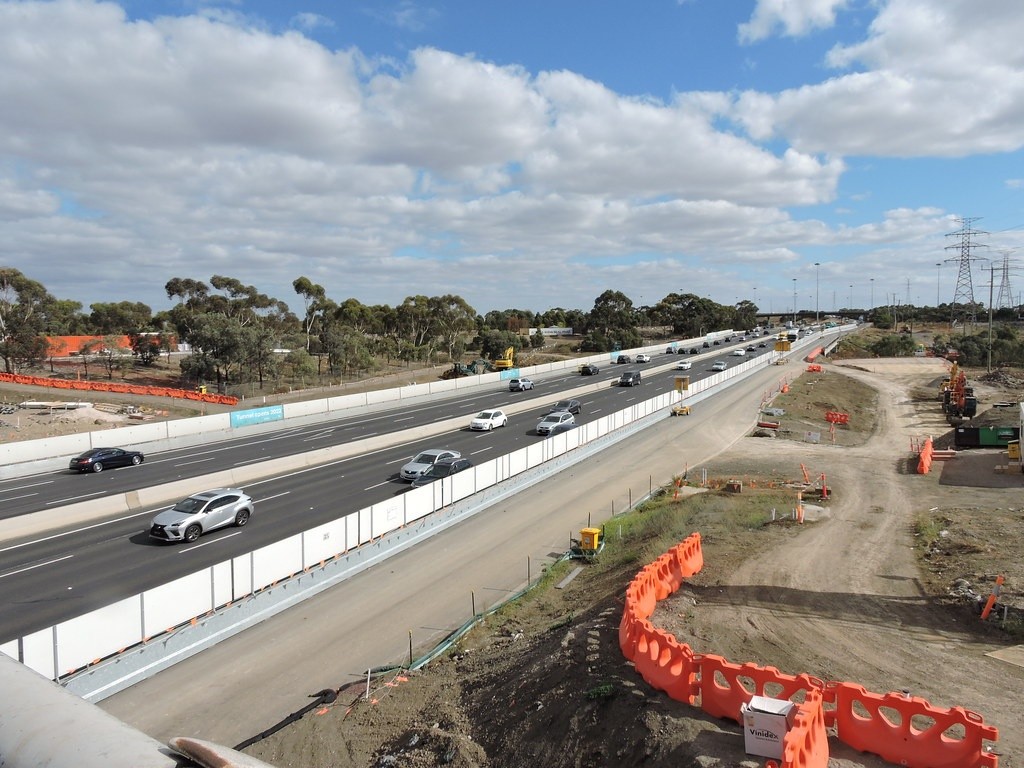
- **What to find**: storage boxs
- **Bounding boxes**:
[741,695,795,758]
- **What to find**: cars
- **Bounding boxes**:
[469,409,508,432]
[148,487,255,544]
[710,314,841,354]
[690,346,702,354]
[676,361,692,370]
[410,458,474,490]
[617,354,631,365]
[733,348,746,357]
[635,354,651,363]
[711,361,727,372]
[702,341,711,348]
[400,448,462,481]
[536,410,576,436]
[548,399,582,415]
[69,446,146,473]
[678,347,689,354]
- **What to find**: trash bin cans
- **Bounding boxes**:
[579,527,601,550]
[918,344,924,352]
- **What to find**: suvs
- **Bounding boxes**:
[509,376,535,392]
[666,346,677,354]
[581,364,600,376]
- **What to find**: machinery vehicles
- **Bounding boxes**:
[936,359,978,425]
[494,346,514,371]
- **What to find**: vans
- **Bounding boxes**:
[618,369,641,387]
